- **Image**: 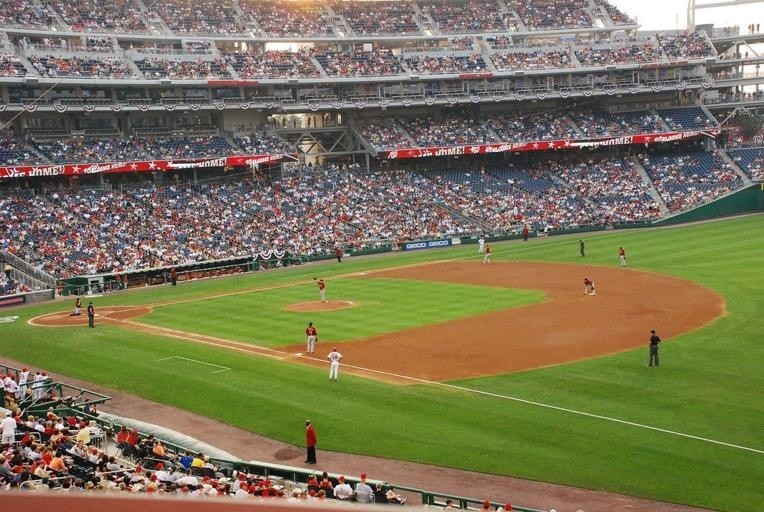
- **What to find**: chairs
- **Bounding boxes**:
[2,0,763,294]
[0,385,266,499]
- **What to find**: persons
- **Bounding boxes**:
[646,330,661,368]
[0,366,387,512]
[305,322,318,353]
[0,0,764,301]
[87,302,96,328]
[74,298,83,315]
[483,500,490,512]
[327,347,344,381]
[505,504,512,512]
[445,499,456,512]
